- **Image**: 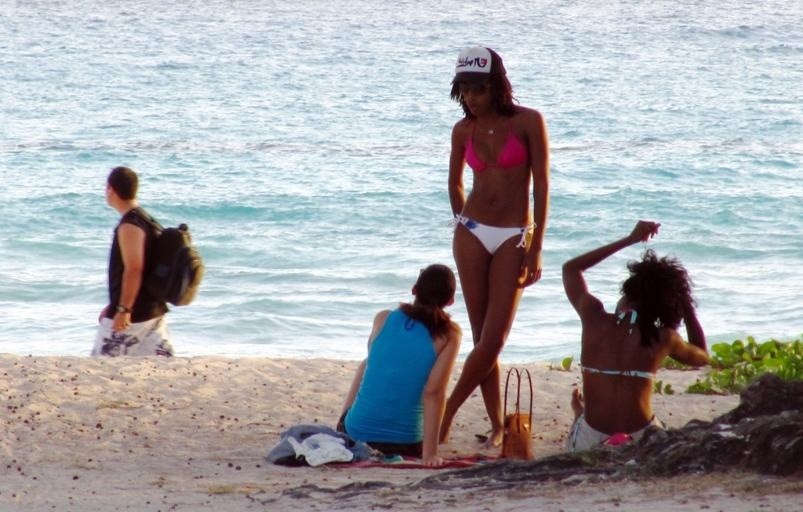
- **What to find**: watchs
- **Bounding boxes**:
[115,304,133,314]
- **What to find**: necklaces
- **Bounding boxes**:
[477,115,503,135]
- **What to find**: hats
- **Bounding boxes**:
[450,46,508,80]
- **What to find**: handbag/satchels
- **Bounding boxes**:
[502,369,537,463]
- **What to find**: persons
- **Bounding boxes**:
[89,167,171,357]
[439,48,548,450]
[337,264,461,468]
[562,220,711,459]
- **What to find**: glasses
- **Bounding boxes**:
[458,82,491,94]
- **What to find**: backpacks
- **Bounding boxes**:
[125,209,203,306]
